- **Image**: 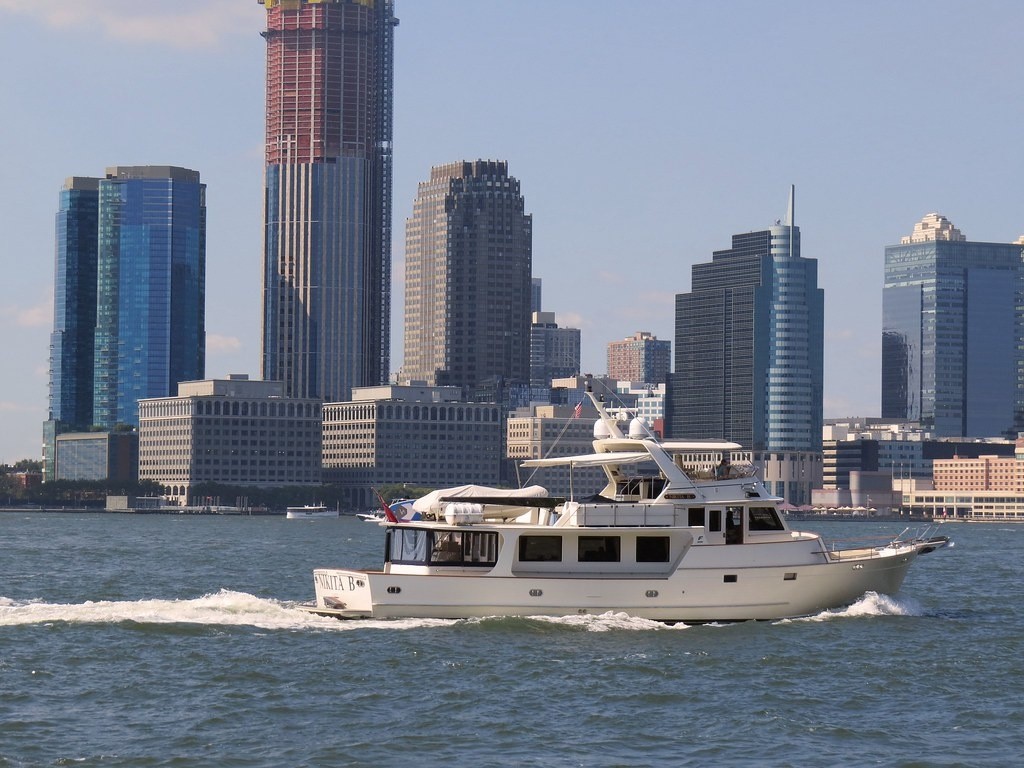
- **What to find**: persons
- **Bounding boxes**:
[726,511,734,545]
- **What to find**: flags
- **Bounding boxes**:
[372,488,398,522]
[575,402,582,420]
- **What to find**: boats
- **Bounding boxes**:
[356,507,388,522]
[293,352,955,626]
[413,483,549,521]
[286,500,340,519]
